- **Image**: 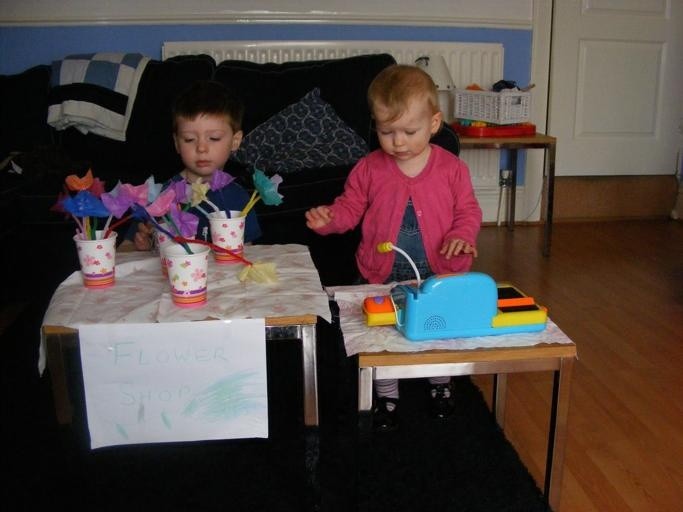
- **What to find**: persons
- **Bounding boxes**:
[115,79,263,254]
[302,62,484,434]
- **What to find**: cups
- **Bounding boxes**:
[163,242,211,308]
[158,220,199,281]
[72,230,119,288]
[207,209,246,266]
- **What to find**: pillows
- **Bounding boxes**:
[228,87,371,181]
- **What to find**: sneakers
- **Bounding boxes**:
[429,383,455,423]
[373,396,401,432]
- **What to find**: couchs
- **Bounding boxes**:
[0,52,461,305]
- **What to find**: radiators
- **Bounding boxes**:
[161,40,503,192]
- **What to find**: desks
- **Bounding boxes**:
[356,281,576,510]
[43,242,324,509]
[456,134,557,258]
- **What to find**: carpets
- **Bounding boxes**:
[1,377,554,510]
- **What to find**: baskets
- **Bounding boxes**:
[452,88,531,125]
